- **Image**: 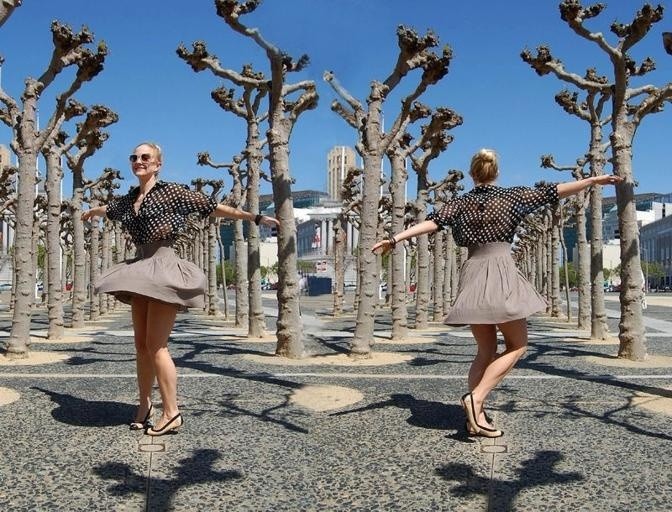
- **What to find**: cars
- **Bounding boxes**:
[1,283,13,292]
[570,287,577,292]
[261,281,279,290]
[603,282,620,292]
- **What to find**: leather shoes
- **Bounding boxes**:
[144,411,185,437]
[130,402,156,431]
[465,421,504,438]
[458,390,485,435]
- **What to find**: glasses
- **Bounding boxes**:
[128,152,157,162]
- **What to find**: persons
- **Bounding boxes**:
[81,141,283,437]
[369,145,626,442]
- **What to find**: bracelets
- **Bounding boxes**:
[392,237,396,248]
[390,236,394,246]
[253,213,263,228]
[388,237,393,246]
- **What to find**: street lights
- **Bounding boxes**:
[642,248,648,292]
